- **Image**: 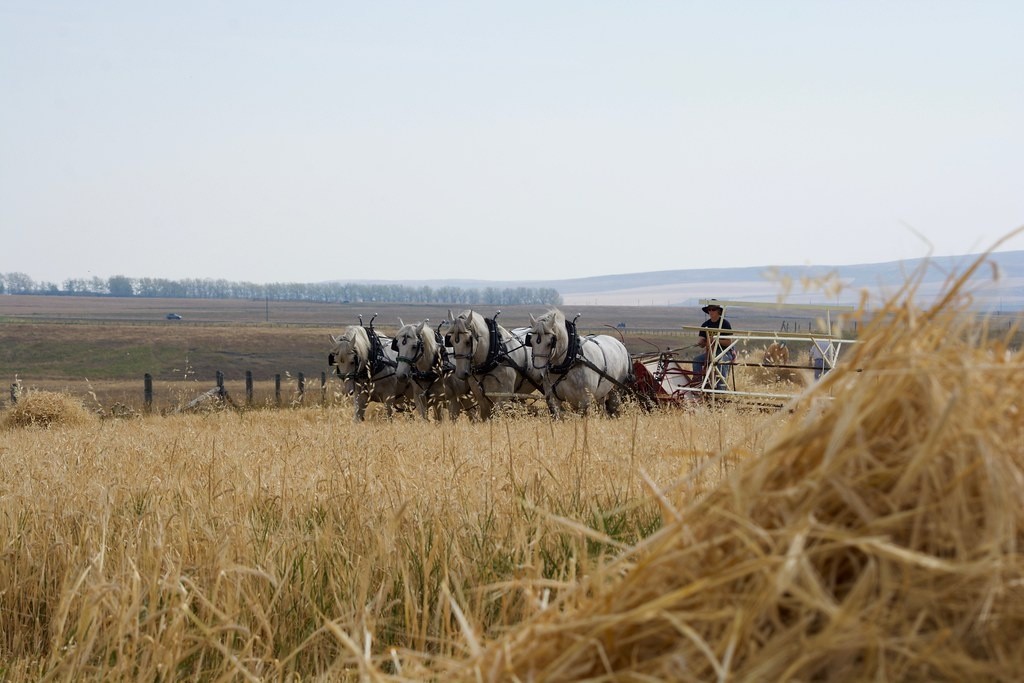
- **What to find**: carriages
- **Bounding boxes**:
[327,306,737,423]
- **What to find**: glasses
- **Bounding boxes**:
[708,308,717,311]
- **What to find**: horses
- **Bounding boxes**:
[328,309,635,420]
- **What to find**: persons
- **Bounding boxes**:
[690,299,734,393]
[809,340,834,380]
[764,340,789,366]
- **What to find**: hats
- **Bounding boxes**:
[702,299,723,316]
